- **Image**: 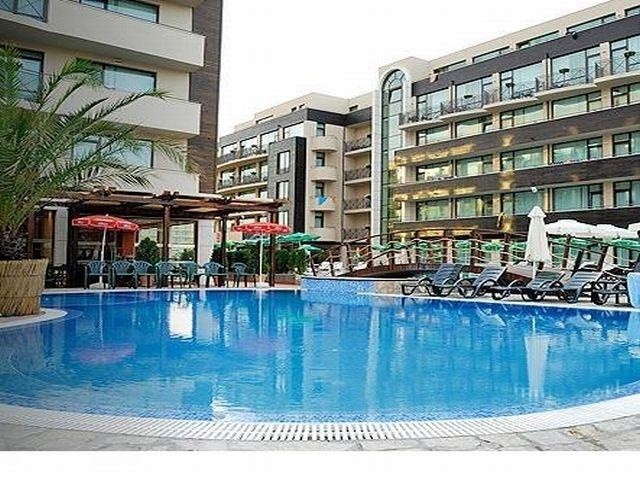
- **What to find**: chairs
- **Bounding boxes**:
[46,260,256,290]
[402,263,640,305]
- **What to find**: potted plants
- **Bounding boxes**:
[0,41,202,316]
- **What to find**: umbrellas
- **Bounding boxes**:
[523,206,640,284]
[71,294,136,365]
[524,317,551,402]
[215,222,325,280]
[230,292,289,354]
[72,213,139,283]
